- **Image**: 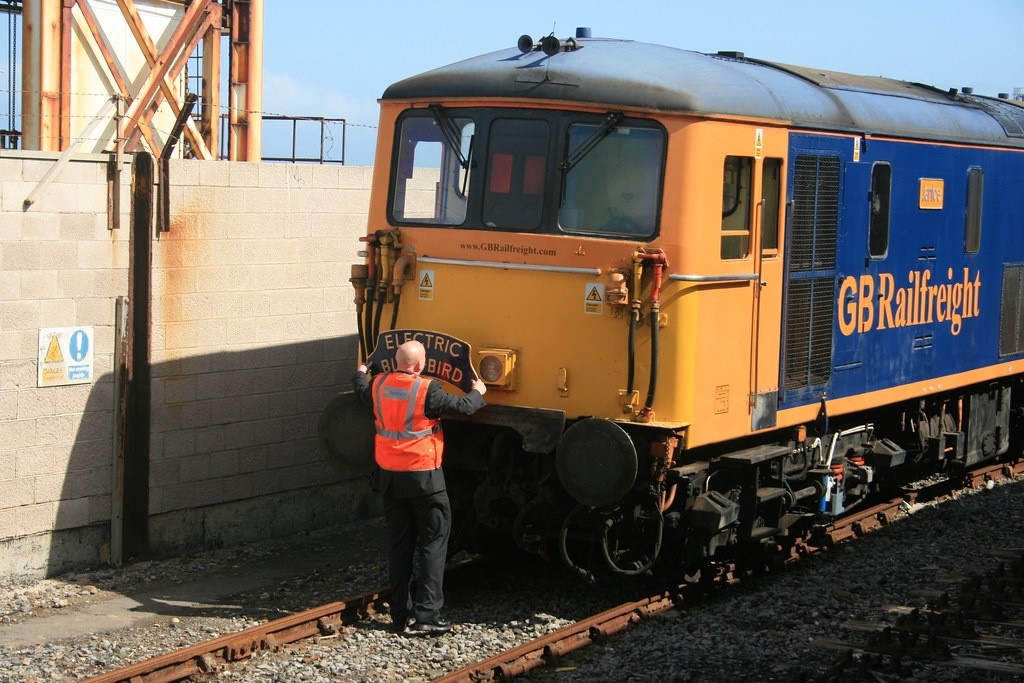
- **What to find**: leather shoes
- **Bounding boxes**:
[410,616,455,630]
[392,616,408,632]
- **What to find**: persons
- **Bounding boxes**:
[352,340,488,630]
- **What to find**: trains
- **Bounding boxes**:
[351,24,1024,594]
